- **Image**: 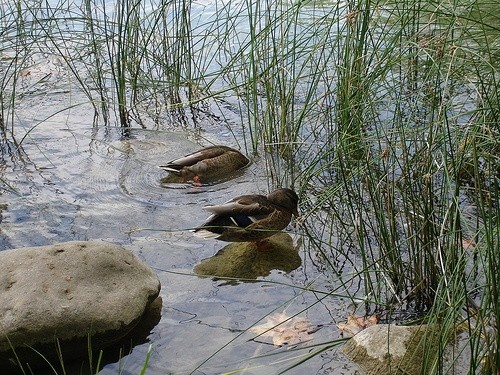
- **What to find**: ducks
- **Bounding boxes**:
[159,145,250,185]
[193,188,299,253]
